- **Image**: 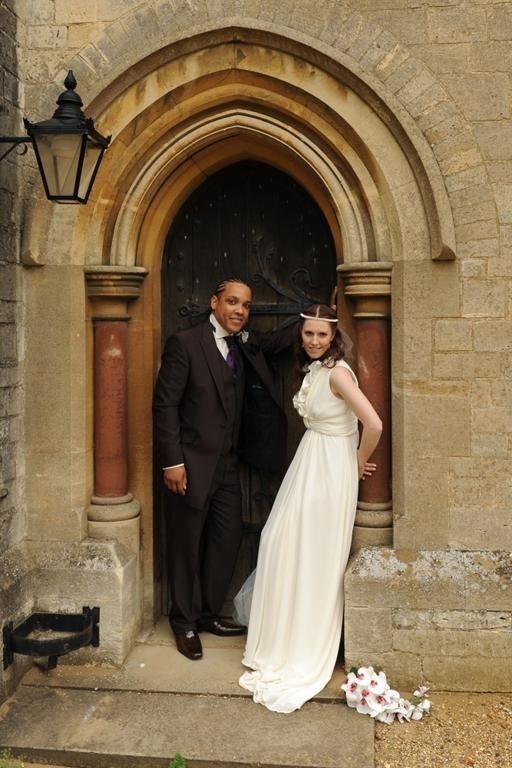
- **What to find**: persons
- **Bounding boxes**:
[232,303,383,714]
[152,277,338,660]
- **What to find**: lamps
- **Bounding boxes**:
[1,70,114,206]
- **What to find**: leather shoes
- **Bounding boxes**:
[171,623,202,659]
[198,618,247,636]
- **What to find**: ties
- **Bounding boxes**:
[223,336,237,369]
